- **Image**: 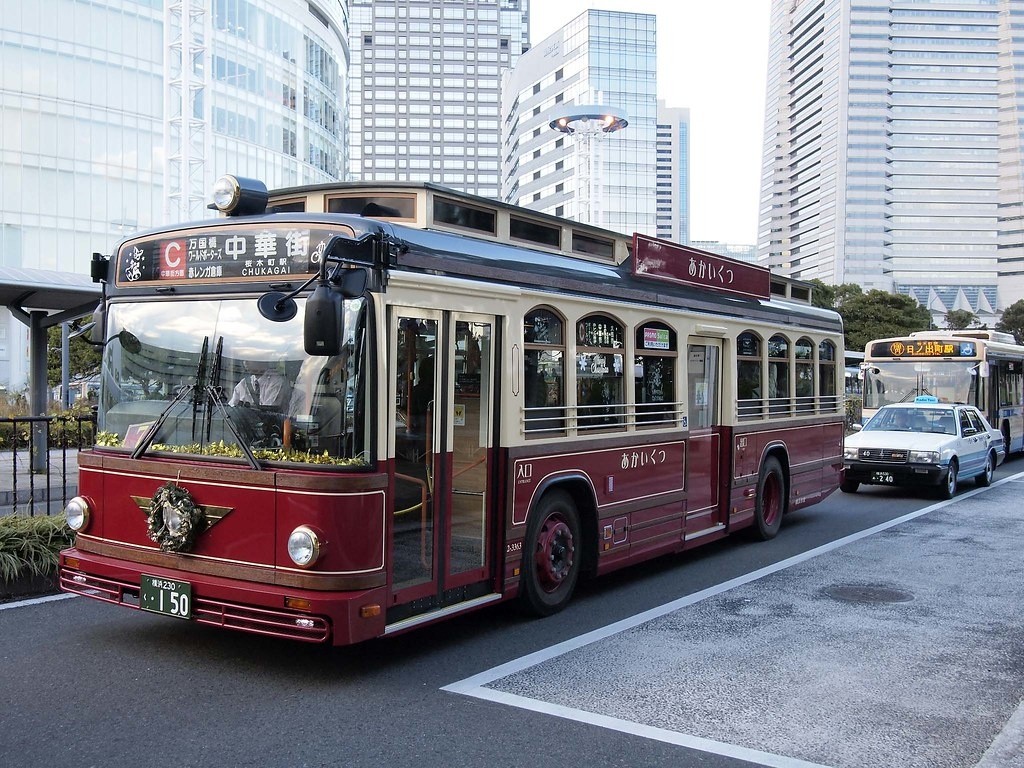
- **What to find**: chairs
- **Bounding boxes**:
[939,417,954,432]
[962,420,979,432]
[912,416,929,427]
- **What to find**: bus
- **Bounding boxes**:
[856,327,1023,465]
[51,172,847,668]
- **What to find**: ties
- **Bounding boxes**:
[253,380,260,405]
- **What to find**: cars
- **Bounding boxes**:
[839,395,1009,497]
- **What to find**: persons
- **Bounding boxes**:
[227,347,291,413]
[539,365,558,377]
[881,412,909,429]
